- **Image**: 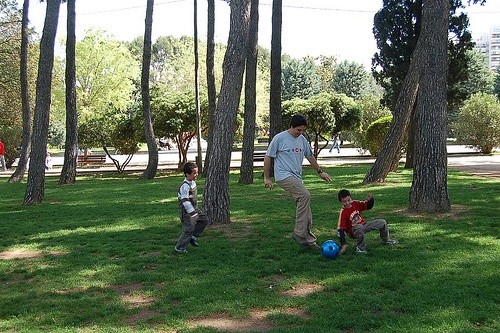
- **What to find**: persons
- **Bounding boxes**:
[173,162,209,255]
[329,134,340,153]
[264,115,332,249]
[45,152,53,170]
[159,141,172,150]
[0,138,7,171]
[337,189,399,255]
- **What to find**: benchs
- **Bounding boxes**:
[78,155,106,167]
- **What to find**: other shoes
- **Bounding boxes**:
[356,247,367,254]
[309,244,320,250]
[189,238,198,246]
[175,247,188,253]
[382,239,396,244]
[292,232,306,244]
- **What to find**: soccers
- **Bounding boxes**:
[321,240,339,259]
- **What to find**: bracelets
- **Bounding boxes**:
[318,169,323,174]
